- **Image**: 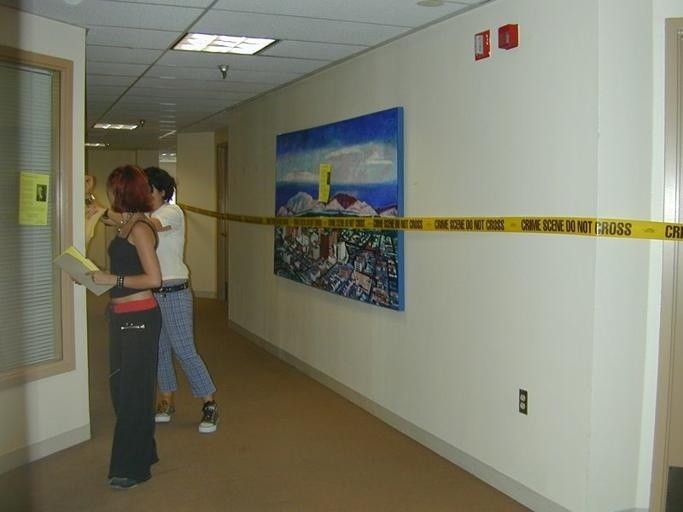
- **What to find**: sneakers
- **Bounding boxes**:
[199,400,219,433]
[155,400,175,423]
[109,471,151,489]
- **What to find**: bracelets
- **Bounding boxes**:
[116,273,126,290]
[84,192,97,206]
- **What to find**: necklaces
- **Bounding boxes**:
[117,212,135,233]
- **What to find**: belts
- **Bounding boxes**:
[152,282,188,293]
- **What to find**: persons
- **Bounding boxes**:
[83,166,222,434]
[68,163,162,489]
